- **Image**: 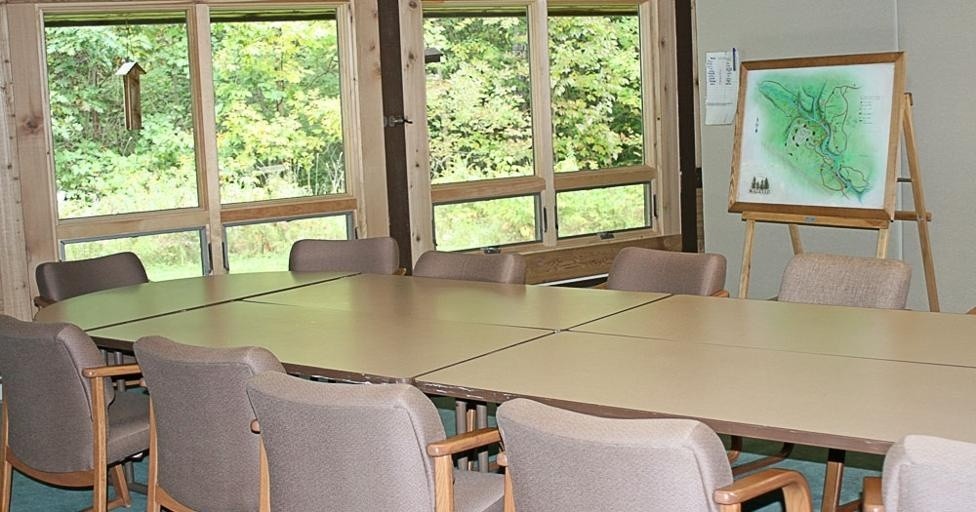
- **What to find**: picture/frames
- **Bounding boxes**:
[726,51,907,223]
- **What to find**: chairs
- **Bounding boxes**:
[1,237,976,512]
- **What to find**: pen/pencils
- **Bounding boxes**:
[733,48,735,70]
